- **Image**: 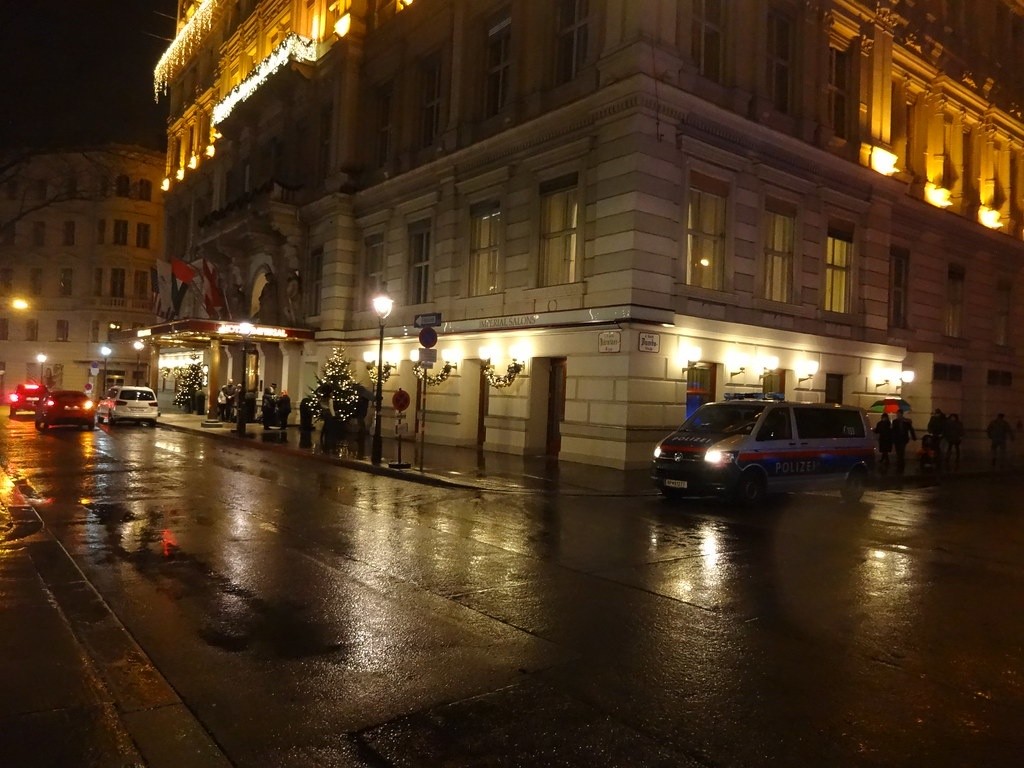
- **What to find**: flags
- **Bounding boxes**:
[202,259,220,313]
[157,259,175,312]
[172,256,194,282]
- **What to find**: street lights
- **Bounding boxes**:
[134,340,145,386]
[236,323,253,435]
[370,281,394,456]
[37,351,46,385]
[101,342,111,396]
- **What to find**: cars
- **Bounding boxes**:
[10,383,95,432]
[98,385,159,426]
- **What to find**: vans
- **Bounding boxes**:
[650,391,881,506]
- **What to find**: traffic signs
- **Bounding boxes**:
[414,312,443,327]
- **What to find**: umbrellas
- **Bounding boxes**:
[869,398,910,413]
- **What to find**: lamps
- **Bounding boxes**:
[896,371,915,390]
[682,346,704,373]
[798,360,820,383]
[441,348,458,371]
[362,351,378,369]
[759,356,779,380]
[876,368,895,387]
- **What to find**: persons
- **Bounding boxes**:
[988,414,1015,466]
[262,383,291,430]
[873,413,891,465]
[892,410,916,472]
[928,409,946,458]
[942,414,964,457]
[218,378,242,422]
